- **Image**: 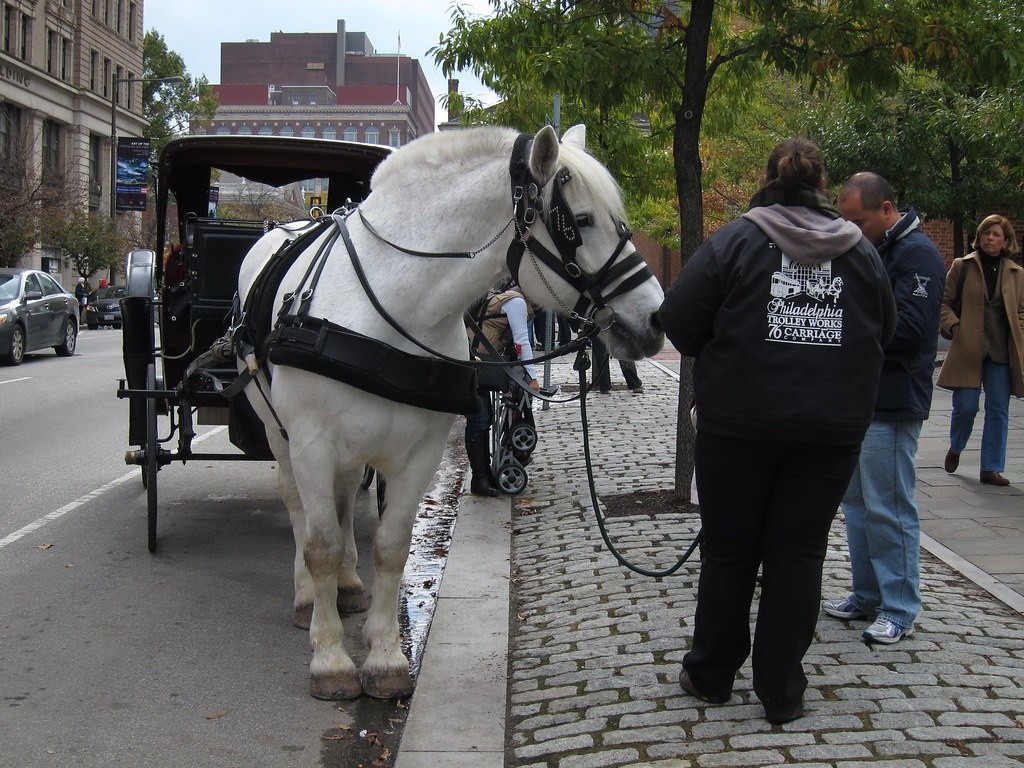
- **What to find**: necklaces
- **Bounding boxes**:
[990,266,998,271]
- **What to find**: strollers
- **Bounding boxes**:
[490,373,559,496]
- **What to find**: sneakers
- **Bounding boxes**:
[980,469,1011,485]
[862,618,915,643]
[821,595,874,618]
[945,447,960,473]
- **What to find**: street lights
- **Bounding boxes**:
[110,74,184,285]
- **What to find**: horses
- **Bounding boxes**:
[234,124,665,702]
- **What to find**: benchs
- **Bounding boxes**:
[182,211,275,321]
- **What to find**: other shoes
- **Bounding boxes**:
[679,667,714,702]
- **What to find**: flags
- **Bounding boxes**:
[397,33,401,51]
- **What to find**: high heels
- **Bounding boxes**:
[470,476,498,497]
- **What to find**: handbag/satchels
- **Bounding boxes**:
[940,259,969,340]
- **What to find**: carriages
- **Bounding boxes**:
[116,124,666,702]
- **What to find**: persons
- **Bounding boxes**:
[939,215,1024,486]
[75,277,104,325]
[465,274,644,498]
[660,138,898,725]
[821,171,946,644]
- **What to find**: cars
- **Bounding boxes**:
[0,268,80,365]
[86,287,159,330]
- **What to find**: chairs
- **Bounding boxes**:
[4,279,30,293]
